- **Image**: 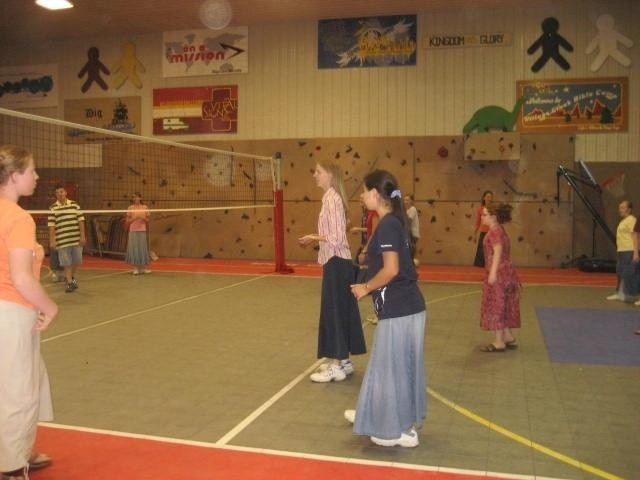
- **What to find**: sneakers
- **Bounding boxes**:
[319,360,354,375]
[607,293,625,301]
[344,409,356,423]
[310,364,347,383]
[50,271,79,293]
[370,426,419,448]
[133,269,152,275]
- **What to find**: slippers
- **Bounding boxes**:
[480,344,506,351]
[28,451,51,469]
[2,465,30,480]
[505,336,517,348]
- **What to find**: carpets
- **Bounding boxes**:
[531,304,640,368]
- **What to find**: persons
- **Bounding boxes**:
[298,156,366,384]
[344,169,428,449]
[607,200,640,305]
[124,193,155,277]
[48,185,86,292]
[350,192,422,269]
[0,145,59,480]
[474,191,495,268]
[473,202,521,352]
[48,247,64,282]
[632,217,640,336]
